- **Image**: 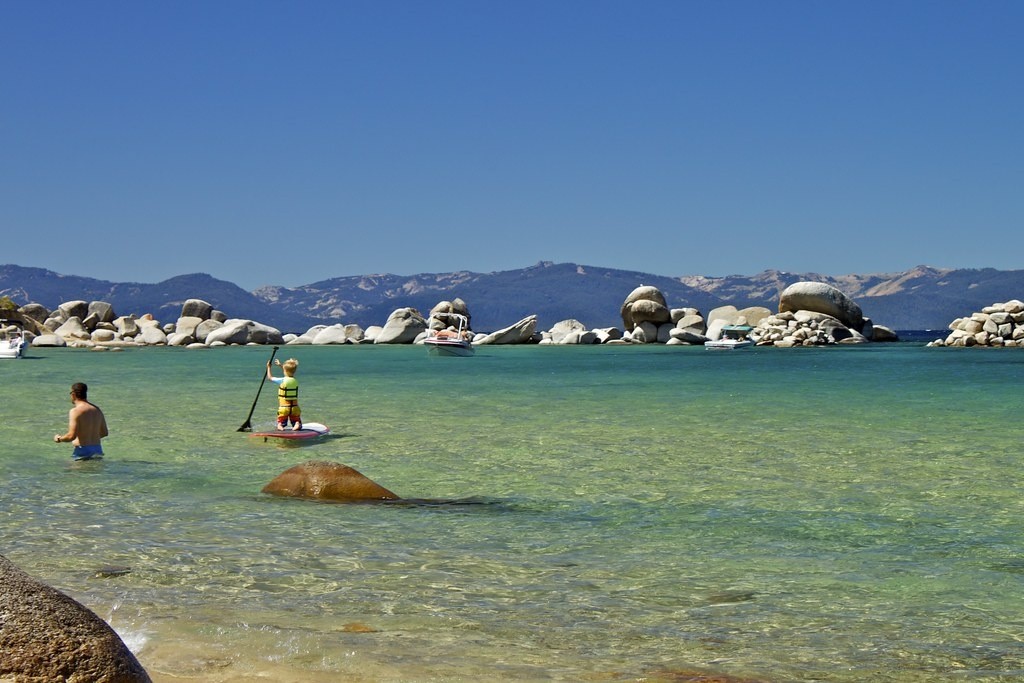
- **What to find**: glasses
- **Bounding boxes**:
[69,390,74,395]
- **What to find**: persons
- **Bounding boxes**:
[54,382,109,462]
[8,333,22,350]
[267,358,303,431]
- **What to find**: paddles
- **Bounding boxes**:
[236,345,280,432]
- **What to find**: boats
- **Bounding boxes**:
[425,313,477,356]
[704,324,753,348]
[0,326,29,360]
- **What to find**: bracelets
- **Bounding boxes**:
[57,435,62,443]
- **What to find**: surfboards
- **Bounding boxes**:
[247,422,330,439]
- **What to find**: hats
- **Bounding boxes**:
[461,325,470,330]
